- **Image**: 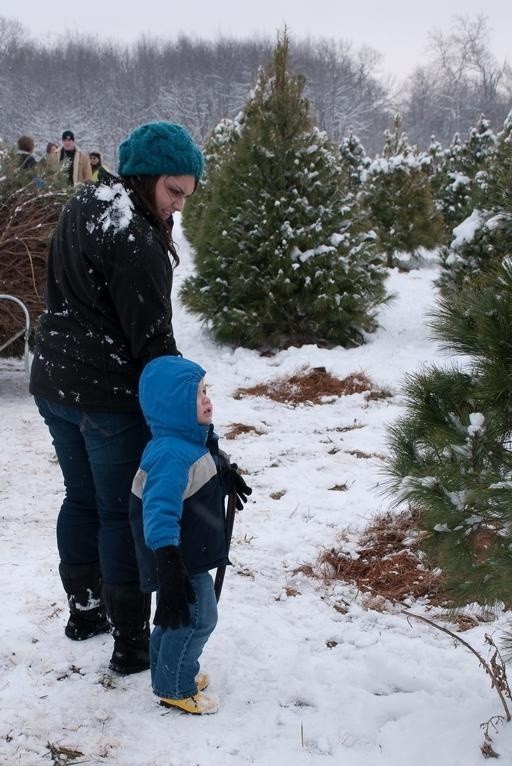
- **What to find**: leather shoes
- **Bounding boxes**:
[158,672,217,715]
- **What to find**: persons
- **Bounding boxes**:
[15,136,36,178]
[26,119,253,675]
[89,149,110,181]
[38,141,57,177]
[50,130,91,187]
[125,356,223,714]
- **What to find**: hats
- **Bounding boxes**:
[118,123,202,180]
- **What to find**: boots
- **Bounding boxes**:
[59,562,113,641]
[102,583,151,674]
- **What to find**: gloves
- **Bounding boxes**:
[154,545,196,630]
[224,463,252,510]
